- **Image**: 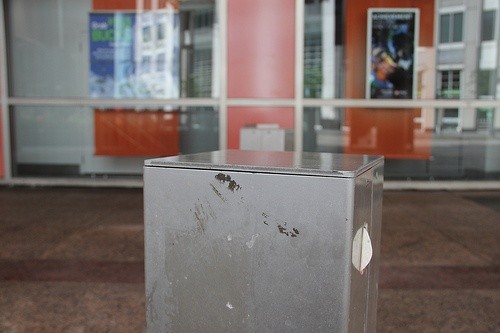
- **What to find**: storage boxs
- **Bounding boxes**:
[141,148,387,332]
